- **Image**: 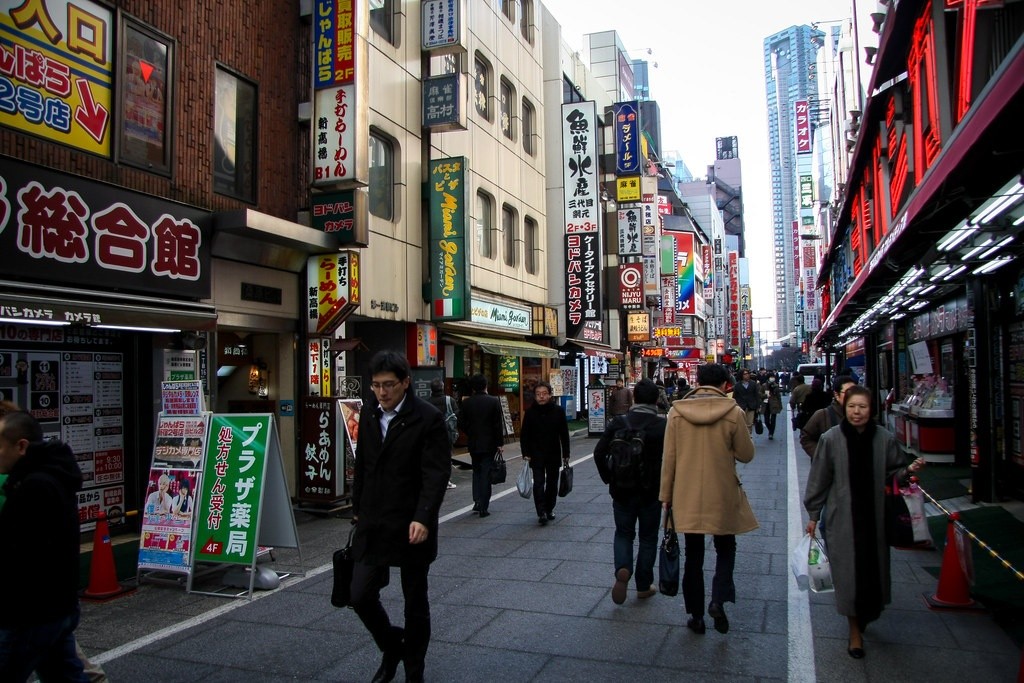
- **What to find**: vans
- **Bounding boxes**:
[795,363,835,392]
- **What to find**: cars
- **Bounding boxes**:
[777,371,794,393]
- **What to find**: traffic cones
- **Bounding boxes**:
[921,511,989,611]
[892,476,936,551]
[78,511,139,605]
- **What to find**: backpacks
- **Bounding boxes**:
[608,416,666,474]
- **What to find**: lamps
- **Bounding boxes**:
[863,47,879,67]
[870,12,887,35]
[854,175,1024,332]
[834,183,846,208]
[629,62,658,68]
[807,17,852,109]
[653,161,675,167]
[621,48,652,55]
[844,111,863,154]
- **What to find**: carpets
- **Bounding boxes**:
[926,504,1024,590]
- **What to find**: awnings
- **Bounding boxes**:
[0,280,217,346]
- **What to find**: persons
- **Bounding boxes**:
[658,363,760,637]
[594,380,666,605]
[424,368,859,546]
[350,351,451,683]
[146,474,194,515]
[0,402,106,683]
[803,385,925,658]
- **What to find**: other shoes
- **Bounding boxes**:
[612,568,630,604]
[769,434,773,439]
[479,510,490,518]
[638,584,657,597]
[447,481,457,488]
[473,503,481,511]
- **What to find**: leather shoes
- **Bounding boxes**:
[847,637,865,658]
[687,618,705,634]
[548,511,556,519]
[538,513,548,523]
[403,656,425,683]
[708,601,729,634]
[372,647,401,683]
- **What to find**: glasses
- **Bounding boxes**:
[371,379,402,393]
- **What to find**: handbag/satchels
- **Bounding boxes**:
[755,411,763,434]
[517,459,533,499]
[883,473,935,549]
[790,531,836,593]
[791,417,796,431]
[659,507,680,597]
[656,396,665,408]
[489,449,506,484]
[771,392,783,414]
[331,524,390,606]
[559,459,574,497]
